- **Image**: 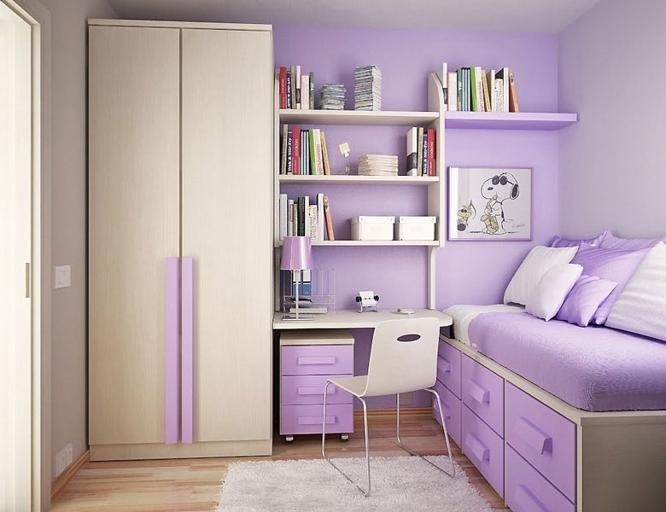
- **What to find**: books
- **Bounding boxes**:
[280,193,335,240]
[281,124,331,175]
[435,62,520,112]
[280,63,383,111]
[406,127,436,176]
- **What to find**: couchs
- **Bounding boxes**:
[432,232,665,511]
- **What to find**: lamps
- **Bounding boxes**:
[278,234,318,323]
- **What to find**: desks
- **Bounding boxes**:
[273,306,453,411]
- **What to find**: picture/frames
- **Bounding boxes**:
[446,164,536,245]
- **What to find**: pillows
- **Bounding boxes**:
[495,234,665,343]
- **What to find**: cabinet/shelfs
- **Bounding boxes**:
[274,65,446,249]
[502,379,580,511]
[433,337,460,452]
[460,352,503,506]
[86,21,275,461]
[277,329,357,443]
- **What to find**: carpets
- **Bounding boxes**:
[216,453,497,511]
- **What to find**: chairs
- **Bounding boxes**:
[321,316,455,498]
